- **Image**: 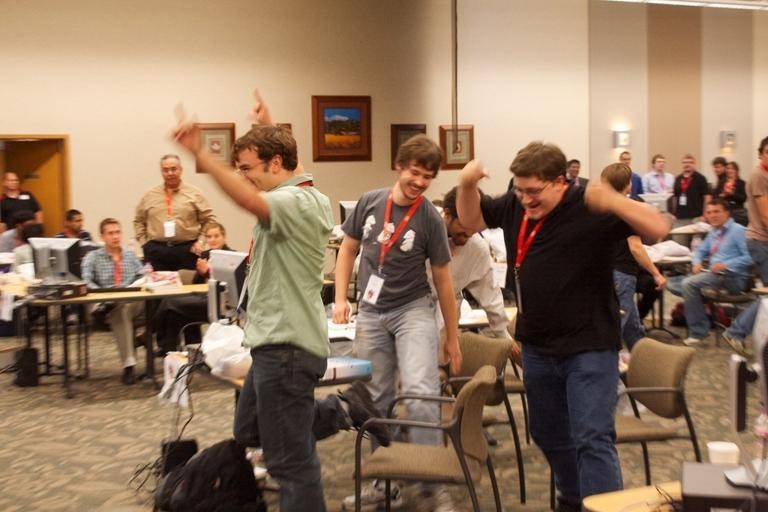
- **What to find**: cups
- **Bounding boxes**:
[707,441,741,465]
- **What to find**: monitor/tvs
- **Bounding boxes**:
[29,237,82,284]
[632,193,678,217]
[339,200,359,225]
[206,248,250,328]
[724,300,768,491]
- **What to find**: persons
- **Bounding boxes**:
[567,137,767,352]
[425,186,518,446]
[175,88,390,512]
[333,136,462,512]
[0,172,232,386]
[457,141,672,511]
[134,156,206,354]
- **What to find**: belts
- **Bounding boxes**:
[151,240,196,248]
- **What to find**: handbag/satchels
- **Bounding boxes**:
[151,437,269,512]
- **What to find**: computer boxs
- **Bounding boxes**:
[27,282,88,301]
[683,462,768,512]
[186,344,211,372]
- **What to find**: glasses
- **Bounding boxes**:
[510,182,552,196]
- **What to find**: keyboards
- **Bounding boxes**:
[88,288,140,293]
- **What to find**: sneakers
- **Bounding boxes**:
[66,313,87,324]
[432,484,454,512]
[341,481,403,511]
[683,335,713,346]
[90,300,116,317]
[336,379,392,449]
[721,328,754,360]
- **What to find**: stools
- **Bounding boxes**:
[166,310,210,360]
[93,309,154,383]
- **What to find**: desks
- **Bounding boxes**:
[210,354,374,407]
[579,457,768,512]
[0,274,68,378]
[24,279,227,398]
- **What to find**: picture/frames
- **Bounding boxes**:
[309,91,376,165]
[387,122,428,172]
[192,121,239,175]
[437,124,476,172]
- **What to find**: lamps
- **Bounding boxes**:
[612,128,632,150]
[718,130,737,150]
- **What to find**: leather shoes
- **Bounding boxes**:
[123,364,137,386]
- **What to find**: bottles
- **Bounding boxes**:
[754,407,768,458]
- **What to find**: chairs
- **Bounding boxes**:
[350,362,504,510]
[224,257,756,341]
[411,329,530,507]
[439,313,531,446]
[602,336,706,485]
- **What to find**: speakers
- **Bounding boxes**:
[160,437,199,480]
[15,345,37,386]
